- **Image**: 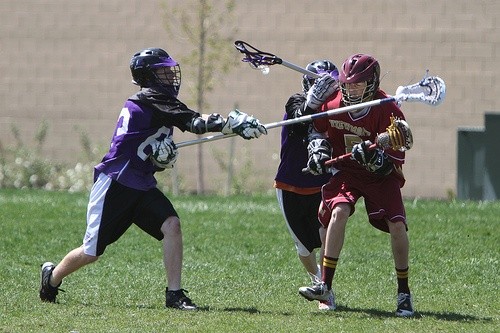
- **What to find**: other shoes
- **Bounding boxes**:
[318,289,337,312]
[307,265,322,287]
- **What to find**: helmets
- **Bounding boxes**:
[304,61,342,90]
[130,48,182,97]
[339,53,381,105]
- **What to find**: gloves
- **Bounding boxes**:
[151,136,178,169]
[351,139,391,175]
[304,74,342,113]
[222,109,268,141]
[308,138,334,177]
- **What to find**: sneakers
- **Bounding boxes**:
[298,280,331,303]
[164,287,197,311]
[395,291,414,317]
[39,261,67,303]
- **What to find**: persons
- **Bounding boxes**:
[40,47,268,309]
[274,59,340,300]
[299,54,414,317]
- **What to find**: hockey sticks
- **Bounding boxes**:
[173,68,446,150]
[302,112,413,173]
[233,40,322,79]
